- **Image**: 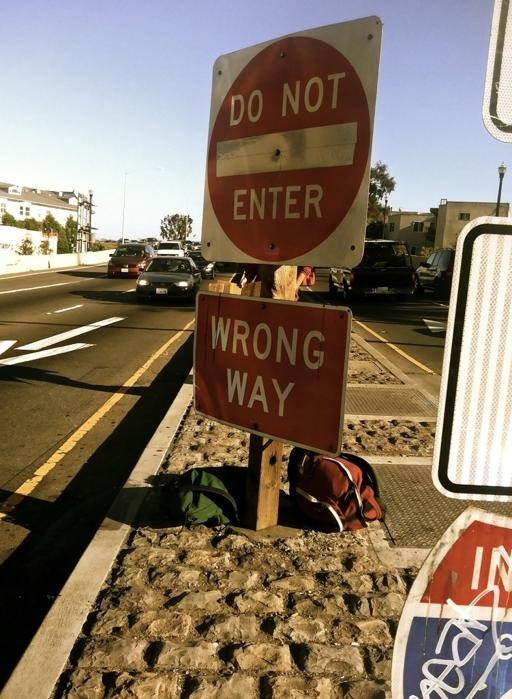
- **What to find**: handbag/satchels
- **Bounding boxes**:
[160,467,252,524]
[288,447,384,532]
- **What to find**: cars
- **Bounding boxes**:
[410,247,455,300]
[136,255,202,303]
[105,237,215,280]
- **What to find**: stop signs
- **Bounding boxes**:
[200,14,382,267]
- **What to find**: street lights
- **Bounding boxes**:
[494,160,507,216]
[88,188,94,250]
[121,171,128,245]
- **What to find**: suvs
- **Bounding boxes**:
[329,236,425,300]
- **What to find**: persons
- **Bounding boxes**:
[136,248,147,257]
[293,266,317,303]
[176,263,192,274]
